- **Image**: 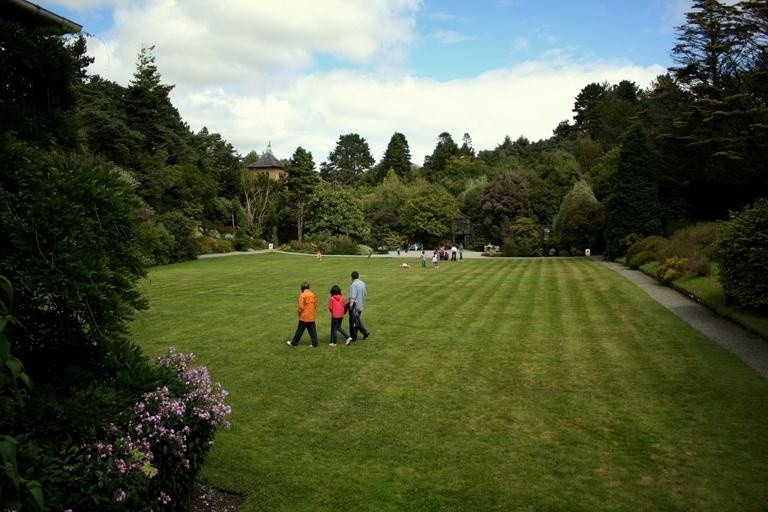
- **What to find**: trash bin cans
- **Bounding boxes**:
[585,249,591,257]
[269,242,274,251]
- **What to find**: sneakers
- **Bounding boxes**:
[345,336,353,345]
[328,342,337,347]
[286,340,295,347]
[308,344,316,348]
[363,331,369,341]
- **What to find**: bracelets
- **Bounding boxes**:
[349,304,352,307]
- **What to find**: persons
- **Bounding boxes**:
[327,284,353,346]
[419,241,464,269]
[311,240,323,261]
[485,242,497,253]
[396,245,401,255]
[286,280,319,348]
[348,270,370,341]
[403,238,409,253]
